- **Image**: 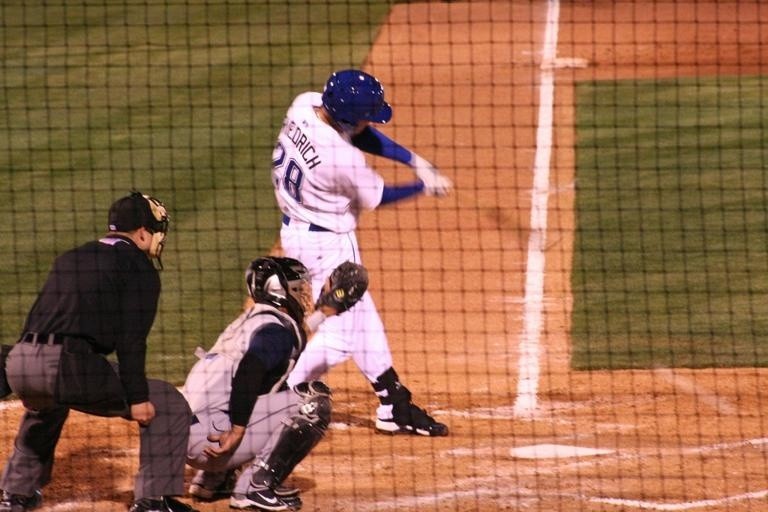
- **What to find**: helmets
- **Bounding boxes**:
[245,255,308,307]
[322,69,392,126]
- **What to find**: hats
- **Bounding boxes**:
[108,195,168,232]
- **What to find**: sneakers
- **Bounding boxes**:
[189,469,303,512]
[129,498,199,512]
[0,489,41,512]
[376,404,448,436]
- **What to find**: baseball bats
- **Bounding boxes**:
[447,187,554,252]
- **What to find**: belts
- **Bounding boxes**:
[18,331,63,344]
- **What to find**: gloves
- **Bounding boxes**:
[405,147,453,196]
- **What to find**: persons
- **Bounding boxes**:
[0,193,195,512]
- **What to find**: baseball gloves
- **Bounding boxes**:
[314,261,369,317]
